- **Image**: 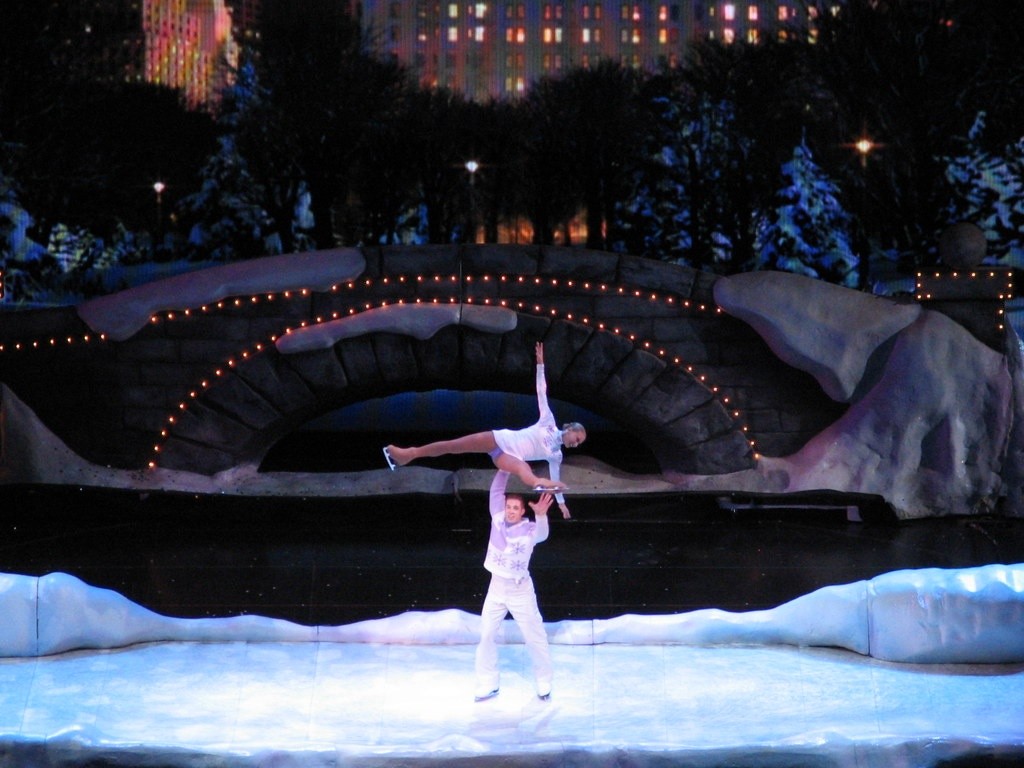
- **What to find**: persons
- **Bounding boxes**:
[474,469,554,703]
[383,342,586,520]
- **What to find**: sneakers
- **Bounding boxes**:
[537,683,551,702]
[383,445,417,471]
[532,478,570,496]
[474,684,500,701]
